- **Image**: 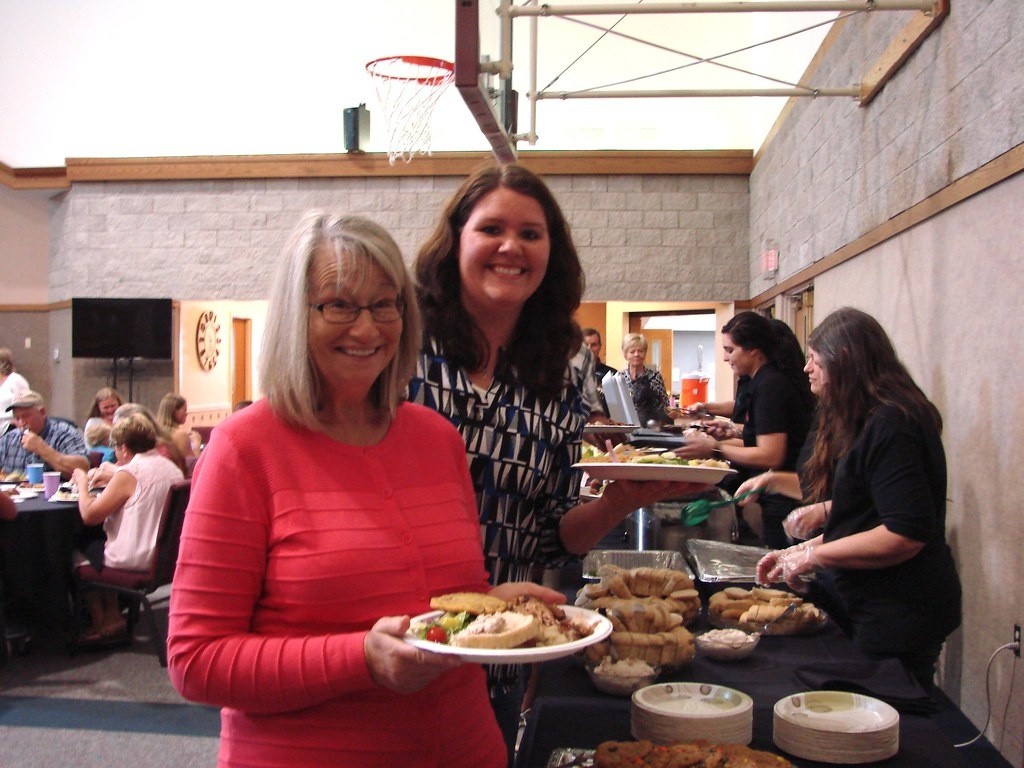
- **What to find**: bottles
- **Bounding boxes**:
[670,394,680,409]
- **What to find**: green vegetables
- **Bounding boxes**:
[416,611,472,641]
[637,457,688,465]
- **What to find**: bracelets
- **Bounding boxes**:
[823,502,828,520]
[712,443,723,458]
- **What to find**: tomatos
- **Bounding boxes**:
[427,628,446,643]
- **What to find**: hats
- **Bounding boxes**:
[4,390,44,412]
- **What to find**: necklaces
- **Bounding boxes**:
[479,366,495,380]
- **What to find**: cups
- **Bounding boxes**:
[43,472,61,499]
[27,464,43,484]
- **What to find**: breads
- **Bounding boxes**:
[575,564,821,668]
[450,610,538,648]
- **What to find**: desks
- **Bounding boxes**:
[505,547,968,768]
[0,481,92,666]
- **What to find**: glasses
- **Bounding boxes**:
[307,299,404,324]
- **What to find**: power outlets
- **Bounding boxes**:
[1013,624,1021,655]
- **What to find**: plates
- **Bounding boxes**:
[773,690,900,763]
[635,447,667,452]
[15,487,45,493]
[584,425,641,433]
[570,462,738,485]
[708,606,828,635]
[48,498,79,502]
[1,480,29,485]
[630,682,754,746]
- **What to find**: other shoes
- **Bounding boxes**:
[79,619,128,641]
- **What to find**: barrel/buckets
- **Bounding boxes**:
[682,378,710,409]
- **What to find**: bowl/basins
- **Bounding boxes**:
[582,660,663,696]
[403,603,613,664]
[578,484,602,502]
[696,635,760,662]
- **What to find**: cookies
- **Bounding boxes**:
[430,592,506,613]
[593,741,792,768]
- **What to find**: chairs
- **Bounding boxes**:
[66,480,197,666]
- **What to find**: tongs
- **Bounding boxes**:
[675,407,710,420]
[681,485,768,527]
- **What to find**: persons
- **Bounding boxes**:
[618,332,674,429]
[232,401,253,414]
[158,392,201,457]
[581,329,618,418]
[674,311,818,551]
[0,387,185,656]
[408,166,708,768]
[734,306,962,682]
[0,346,30,438]
[569,344,627,452]
[166,210,566,768]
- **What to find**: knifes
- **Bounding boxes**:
[732,603,797,649]
[88,483,95,492]
